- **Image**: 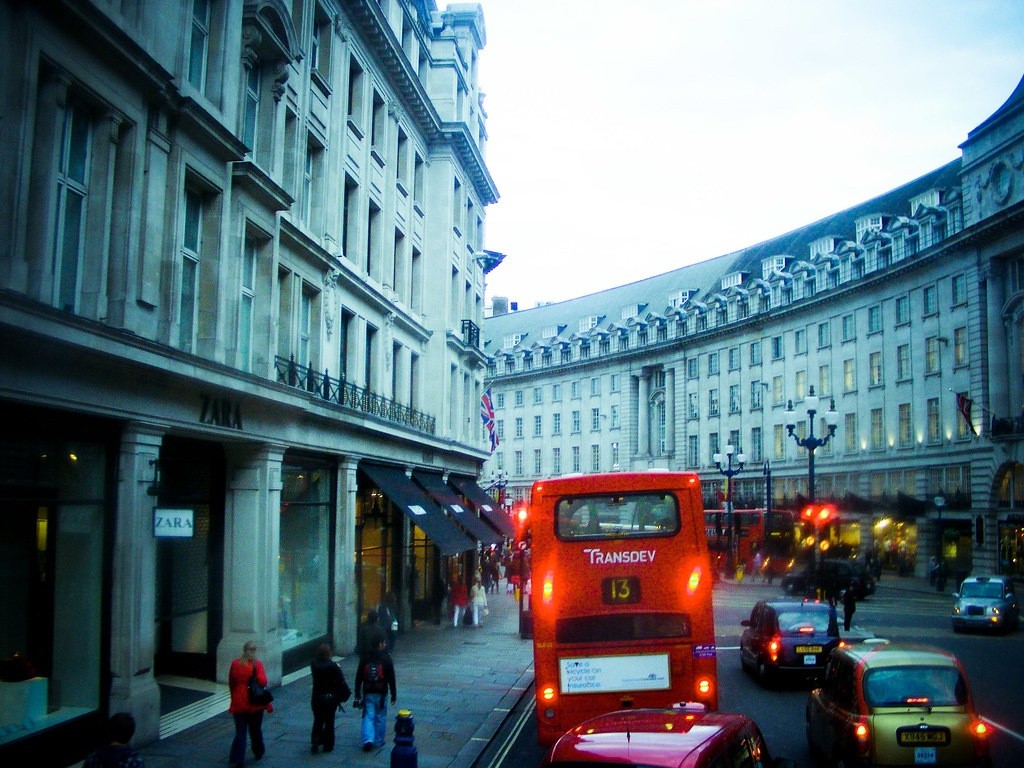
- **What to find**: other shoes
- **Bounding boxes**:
[312,746,318,753]
[364,743,372,752]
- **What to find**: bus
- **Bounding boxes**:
[531,464,721,747]
[704,510,794,554]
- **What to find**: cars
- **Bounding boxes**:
[950,574,1019,633]
[805,635,999,768]
[740,596,845,690]
[548,706,775,768]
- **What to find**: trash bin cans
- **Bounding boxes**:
[522,592,533,639]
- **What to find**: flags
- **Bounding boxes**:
[954,394,977,435]
[716,488,726,502]
[481,386,499,451]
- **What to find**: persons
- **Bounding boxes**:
[353,633,398,752]
[227,640,273,768]
[450,573,468,628]
[752,549,762,583]
[379,590,399,655]
[82,712,145,768]
[310,642,353,755]
[479,531,531,593]
[469,572,489,628]
[356,611,383,655]
[928,555,940,587]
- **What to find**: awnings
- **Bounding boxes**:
[358,461,518,555]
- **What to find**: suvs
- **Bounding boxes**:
[781,558,877,603]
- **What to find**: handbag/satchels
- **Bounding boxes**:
[247,661,274,705]
[391,621,398,631]
[463,607,472,625]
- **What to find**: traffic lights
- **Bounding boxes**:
[803,504,835,525]
[518,507,527,522]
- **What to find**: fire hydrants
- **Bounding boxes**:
[391,710,419,768]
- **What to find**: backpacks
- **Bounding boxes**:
[363,663,384,692]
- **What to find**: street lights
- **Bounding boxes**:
[934,490,948,591]
[490,465,509,507]
[763,459,772,516]
[713,446,747,579]
[784,385,839,504]
